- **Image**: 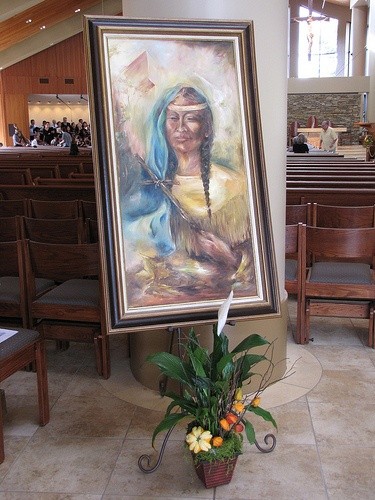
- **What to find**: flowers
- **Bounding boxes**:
[145,291,278,475]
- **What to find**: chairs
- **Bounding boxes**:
[287,152,375,350]
[289,122,300,146]
[306,116,318,128]
[1,150,110,379]
[0,326,50,463]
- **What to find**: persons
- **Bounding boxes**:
[30,132,46,147]
[34,117,91,147]
[319,121,337,153]
[30,120,39,135]
[13,129,31,147]
[293,132,309,153]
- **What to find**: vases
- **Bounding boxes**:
[191,447,243,489]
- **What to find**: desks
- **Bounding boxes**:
[297,128,347,148]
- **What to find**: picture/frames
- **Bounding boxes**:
[83,14,281,336]
